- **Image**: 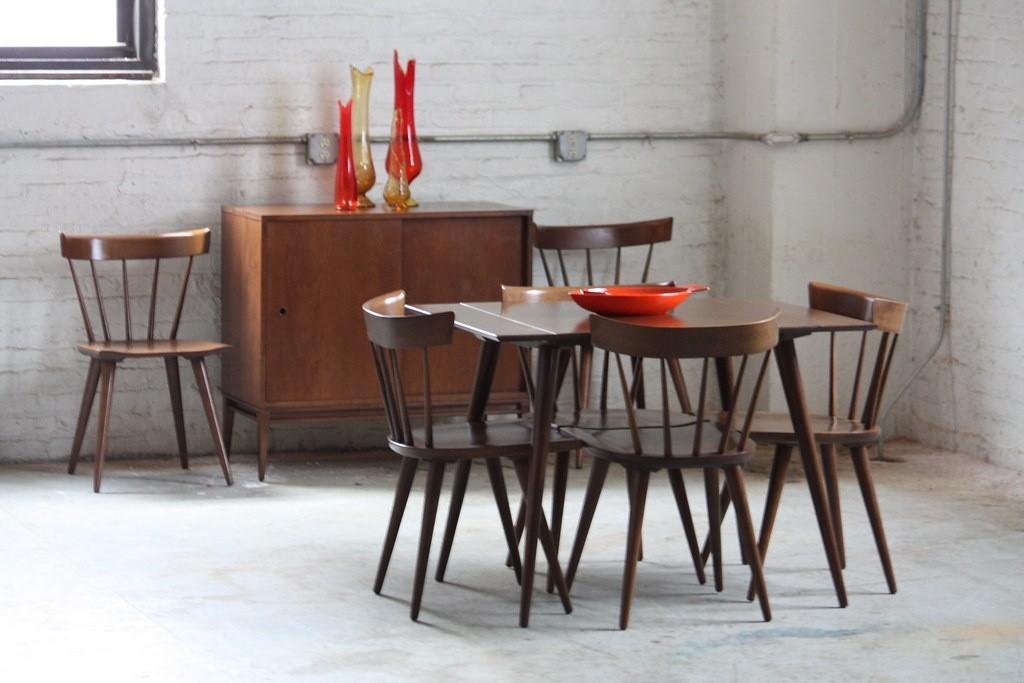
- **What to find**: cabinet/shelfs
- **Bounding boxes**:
[219,203,537,483]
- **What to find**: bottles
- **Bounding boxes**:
[349,64,376,209]
[383,109,410,212]
[335,99,357,210]
[385,47,422,206]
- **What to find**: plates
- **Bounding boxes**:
[567,285,712,315]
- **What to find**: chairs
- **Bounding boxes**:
[500,278,710,596]
[697,280,912,604]
[55,230,234,491]
[557,311,783,632]
[531,214,691,467]
[357,290,576,626]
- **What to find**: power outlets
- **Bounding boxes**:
[306,132,340,165]
[555,130,588,162]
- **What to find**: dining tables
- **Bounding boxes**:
[408,290,852,632]
